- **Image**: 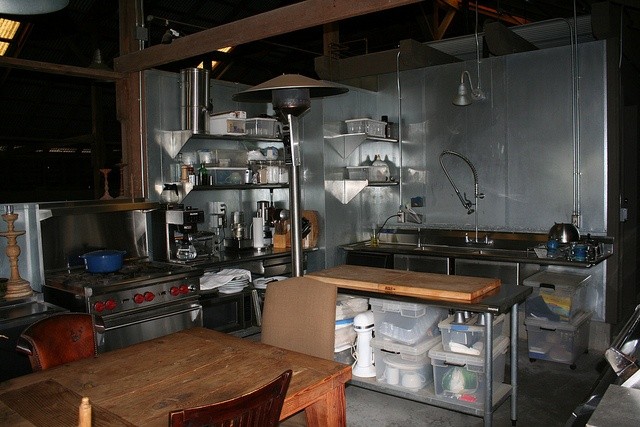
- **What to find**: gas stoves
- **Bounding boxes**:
[56,260,186,288]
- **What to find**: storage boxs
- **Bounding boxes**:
[523,311,590,370]
[335,320,356,352]
[346,165,390,183]
[197,167,249,186]
[428,335,510,407]
[334,347,356,365]
[227,118,277,138]
[437,314,504,356]
[369,297,450,346]
[370,334,442,393]
[336,294,368,321]
[523,270,592,324]
[344,119,387,138]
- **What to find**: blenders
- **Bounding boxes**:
[351,311,375,378]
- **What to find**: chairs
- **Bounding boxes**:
[17,313,104,372]
[169,369,293,426]
[260,277,338,362]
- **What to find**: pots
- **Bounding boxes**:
[78,249,128,273]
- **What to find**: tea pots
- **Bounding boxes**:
[160,183,179,202]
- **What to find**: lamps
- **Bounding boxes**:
[231,71,350,275]
[452,1,488,107]
[163,20,173,45]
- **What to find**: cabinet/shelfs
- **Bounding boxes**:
[161,133,294,256]
[0,57,162,215]
[343,133,399,186]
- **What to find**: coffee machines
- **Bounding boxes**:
[165,202,205,265]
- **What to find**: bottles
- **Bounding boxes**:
[78,397,92,427]
[198,162,208,186]
[266,160,279,184]
[257,160,266,185]
[175,153,183,182]
[276,160,288,184]
